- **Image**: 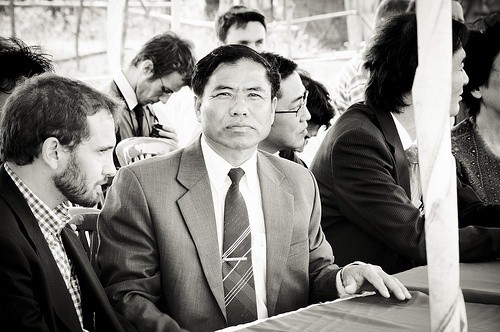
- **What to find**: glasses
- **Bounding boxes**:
[275,91,309,118]
[160,78,173,94]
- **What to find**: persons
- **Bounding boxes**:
[451,10,500,206]
[309,11,500,275]
[288,0,465,152]
[97,31,196,209]
[258,51,311,168]
[216,6,267,54]
[0,36,55,111]
[96,44,412,331]
[0,72,124,332]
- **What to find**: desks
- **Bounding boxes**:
[216,262,500,332]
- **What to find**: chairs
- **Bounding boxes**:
[115,136,179,168]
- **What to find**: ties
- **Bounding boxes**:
[133,105,144,137]
[221,167,258,326]
[404,147,422,208]
